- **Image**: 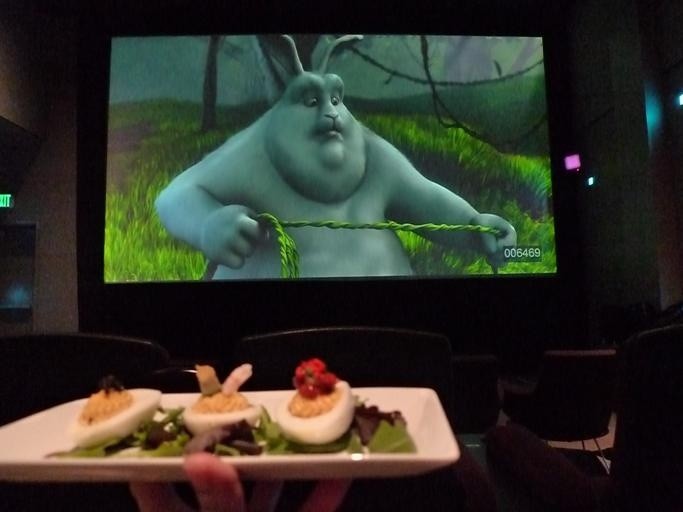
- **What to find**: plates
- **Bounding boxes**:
[0,385,462,484]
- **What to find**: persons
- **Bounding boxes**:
[126,449,351,511]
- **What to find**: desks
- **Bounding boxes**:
[2,384,462,511]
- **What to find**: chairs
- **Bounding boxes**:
[452,352,499,431]
[15,329,175,386]
[481,326,683,512]
[242,328,451,386]
[499,348,614,457]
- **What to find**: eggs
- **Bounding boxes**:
[184,390,260,438]
[277,379,355,445]
[66,389,160,445]
